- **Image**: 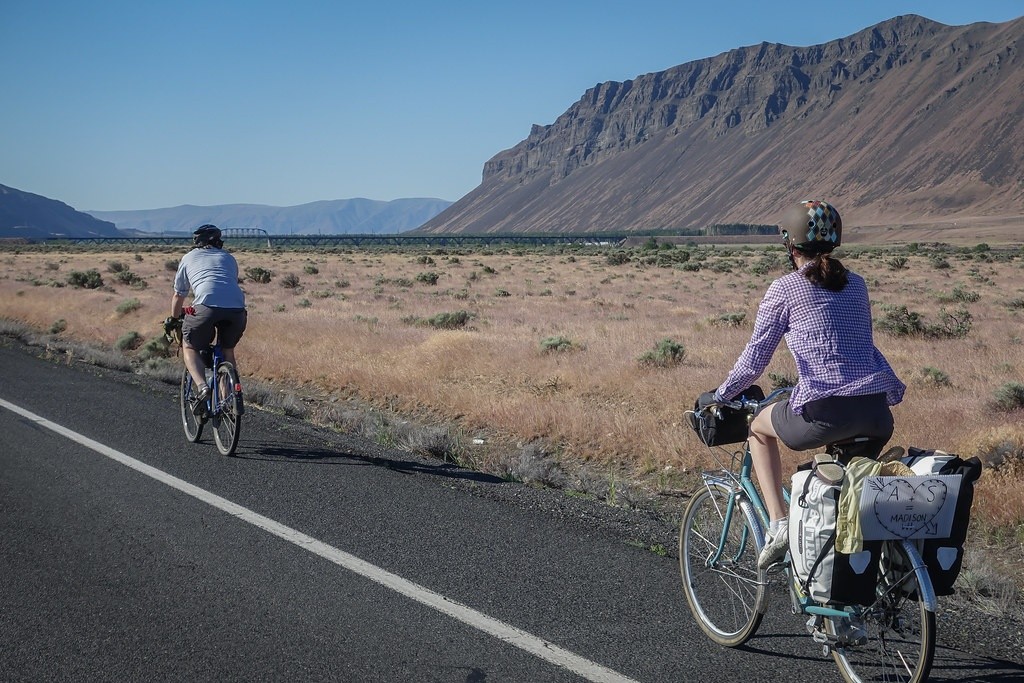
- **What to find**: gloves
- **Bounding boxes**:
[164,314,179,333]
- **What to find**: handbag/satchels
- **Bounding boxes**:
[785,448,983,608]
[687,383,766,447]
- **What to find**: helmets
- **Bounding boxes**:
[780,199,843,250]
[192,224,222,243]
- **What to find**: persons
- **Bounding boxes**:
[697,199,907,645]
[162,224,249,423]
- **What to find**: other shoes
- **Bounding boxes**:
[810,452,845,487]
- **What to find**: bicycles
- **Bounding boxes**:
[678,393,938,683]
[164,316,244,456]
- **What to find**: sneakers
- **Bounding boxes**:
[190,385,211,415]
[756,518,790,568]
